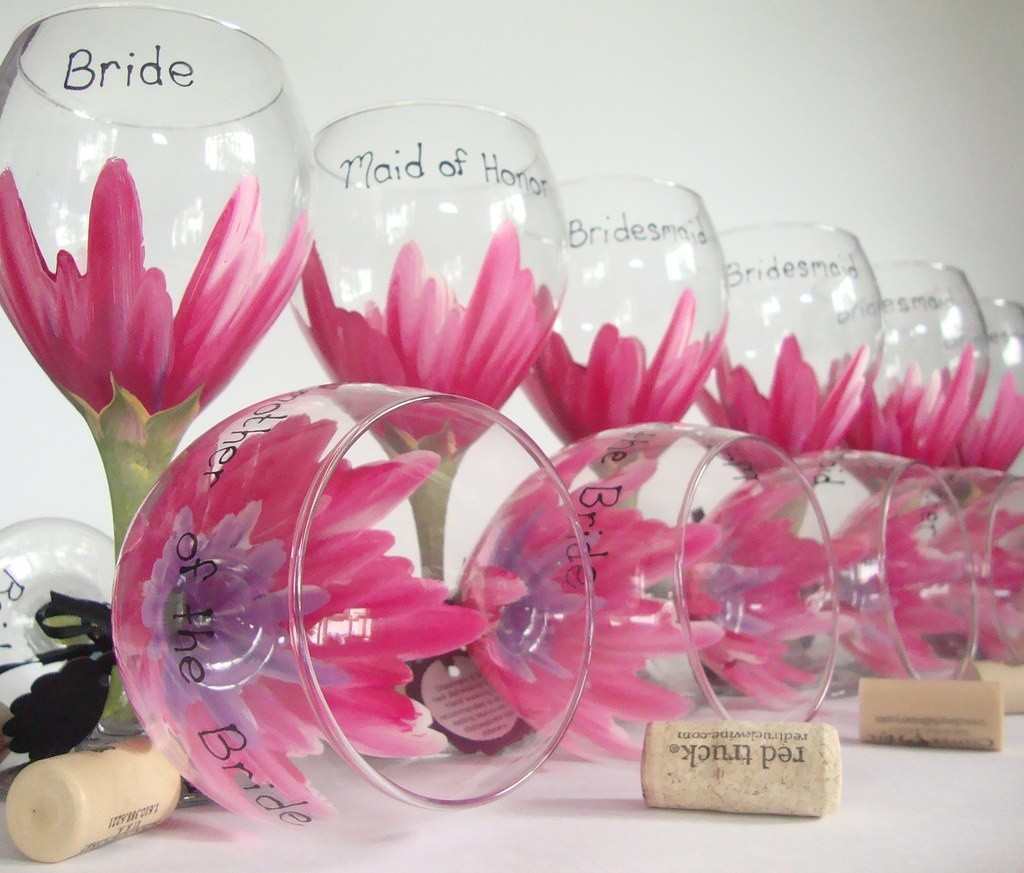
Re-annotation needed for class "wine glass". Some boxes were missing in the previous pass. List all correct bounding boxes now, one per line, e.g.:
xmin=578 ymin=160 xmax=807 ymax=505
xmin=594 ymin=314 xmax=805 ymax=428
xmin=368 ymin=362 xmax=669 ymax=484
xmin=872 ymin=262 xmax=989 ymax=469
xmin=696 ymin=223 xmax=886 ymax=427
xmin=1 ymin=7 xmax=321 ymax=808
xmin=521 ymin=174 xmax=729 ymax=444
xmin=935 ymin=468 xmax=1024 ymax=668
xmin=549 ymin=422 xmax=838 ymax=766
xmin=979 ymin=300 xmax=1024 ymax=472
xmin=792 ymin=450 xmax=977 ymax=700
xmin=291 ymin=103 xmax=568 ymax=412
xmin=2 ymin=382 xmax=595 ymax=842
